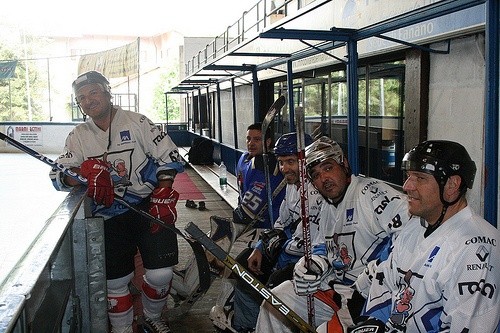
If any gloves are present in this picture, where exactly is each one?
[291,253,329,296]
[148,187,180,235]
[258,228,288,251]
[79,158,114,207]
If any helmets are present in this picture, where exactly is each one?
[72,70,109,92]
[273,132,314,156]
[401,140,477,189]
[305,136,344,181]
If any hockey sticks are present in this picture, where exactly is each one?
[261,95,286,230]
[184,220,318,333]
[240,128,329,234]
[0,131,211,290]
[295,107,316,330]
[161,121,328,321]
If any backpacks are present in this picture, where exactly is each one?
[184,138,214,165]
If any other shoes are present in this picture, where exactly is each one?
[142,313,172,333]
[186,200,197,208]
[209,306,255,333]
[199,201,205,210]
[171,268,190,297]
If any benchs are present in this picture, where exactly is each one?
[167,129,247,210]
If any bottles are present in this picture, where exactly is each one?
[219,162,229,192]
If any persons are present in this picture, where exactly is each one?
[347,140,500,333]
[254,135,412,333]
[169,122,324,333]
[50,71,186,333]
